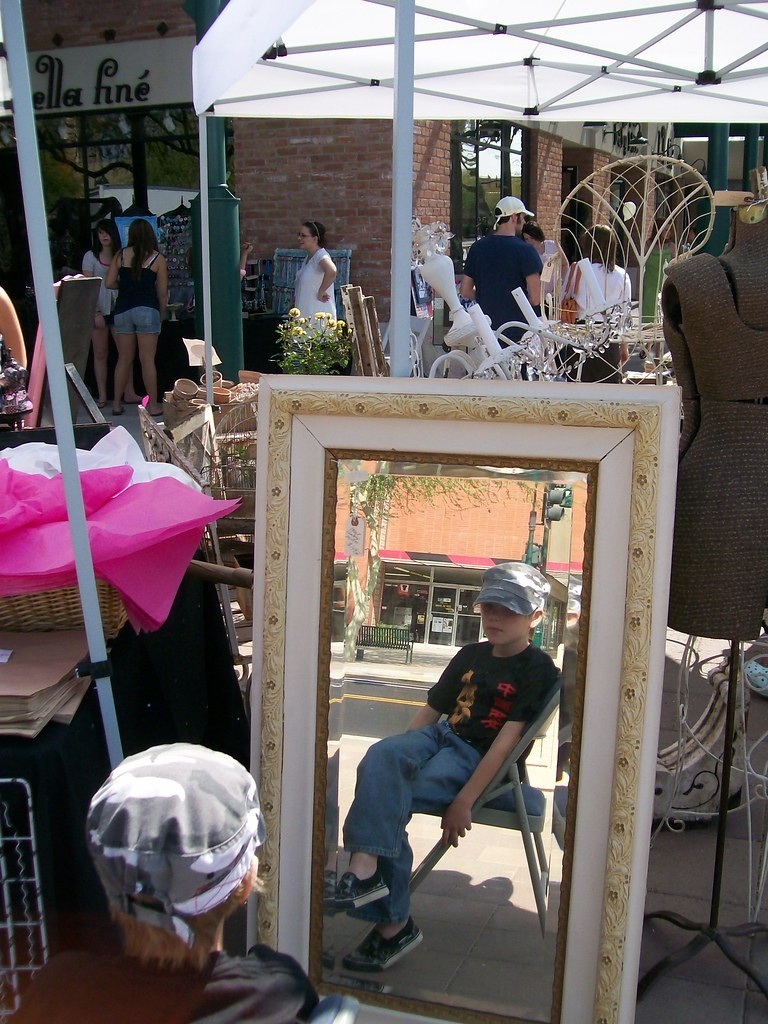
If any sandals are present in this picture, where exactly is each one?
[744,660,768,697]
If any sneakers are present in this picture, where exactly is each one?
[342,913,424,972]
[322,865,391,913]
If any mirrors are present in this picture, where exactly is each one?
[255,368,686,1024]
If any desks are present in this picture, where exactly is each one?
[89,314,283,404]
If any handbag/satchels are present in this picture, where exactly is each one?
[561,262,581,323]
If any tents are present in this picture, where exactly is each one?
[186,1,768,402]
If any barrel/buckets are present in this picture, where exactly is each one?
[357,649,364,659]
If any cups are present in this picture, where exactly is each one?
[645,361,653,372]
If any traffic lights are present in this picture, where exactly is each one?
[542,486,567,521]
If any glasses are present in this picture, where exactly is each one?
[297,233,314,238]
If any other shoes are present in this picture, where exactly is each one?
[112,407,123,415]
[149,410,163,416]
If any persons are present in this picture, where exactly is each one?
[0,742,319,1024]
[331,562,559,974]
[240,242,255,282]
[0,286,27,395]
[558,225,632,385]
[521,221,564,303]
[460,196,544,348]
[82,219,148,408]
[290,220,338,374]
[638,218,676,359]
[104,217,168,417]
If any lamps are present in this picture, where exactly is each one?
[653,143,687,165]
[582,121,607,129]
[681,158,707,175]
[409,217,634,382]
[602,122,647,146]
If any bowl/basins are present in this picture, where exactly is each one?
[171,371,235,405]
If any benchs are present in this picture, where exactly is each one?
[347,622,418,666]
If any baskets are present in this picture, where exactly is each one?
[0,578,130,641]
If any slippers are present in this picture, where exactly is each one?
[125,400,149,404]
[97,401,105,408]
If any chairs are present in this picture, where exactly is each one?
[341,281,431,376]
[411,684,562,942]
[552,155,711,386]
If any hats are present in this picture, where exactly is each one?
[472,563,552,615]
[84,743,269,949]
[494,196,535,230]
